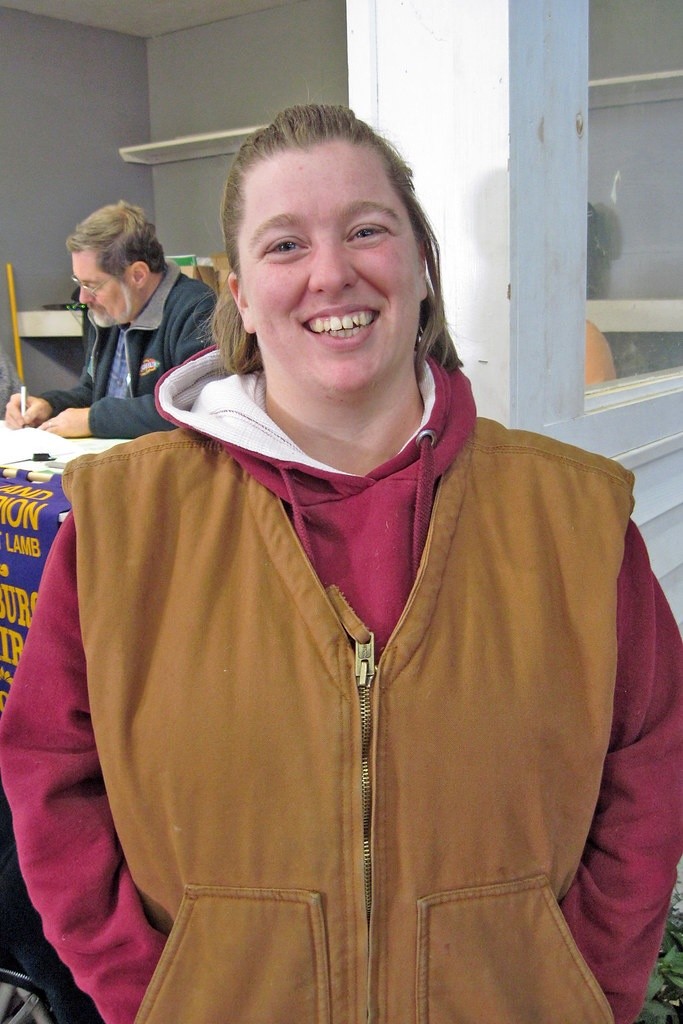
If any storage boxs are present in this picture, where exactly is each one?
[211,253,230,291]
[177,263,217,294]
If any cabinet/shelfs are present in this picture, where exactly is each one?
[17,68,683,339]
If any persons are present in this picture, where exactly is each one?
[0,102,683,1024]
[0,347,28,421]
[5,202,221,439]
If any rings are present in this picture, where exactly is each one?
[49,421,52,428]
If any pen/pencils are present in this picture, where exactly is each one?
[20,384,26,428]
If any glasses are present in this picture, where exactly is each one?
[70,272,116,297]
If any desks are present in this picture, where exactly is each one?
[0,437,135,715]
